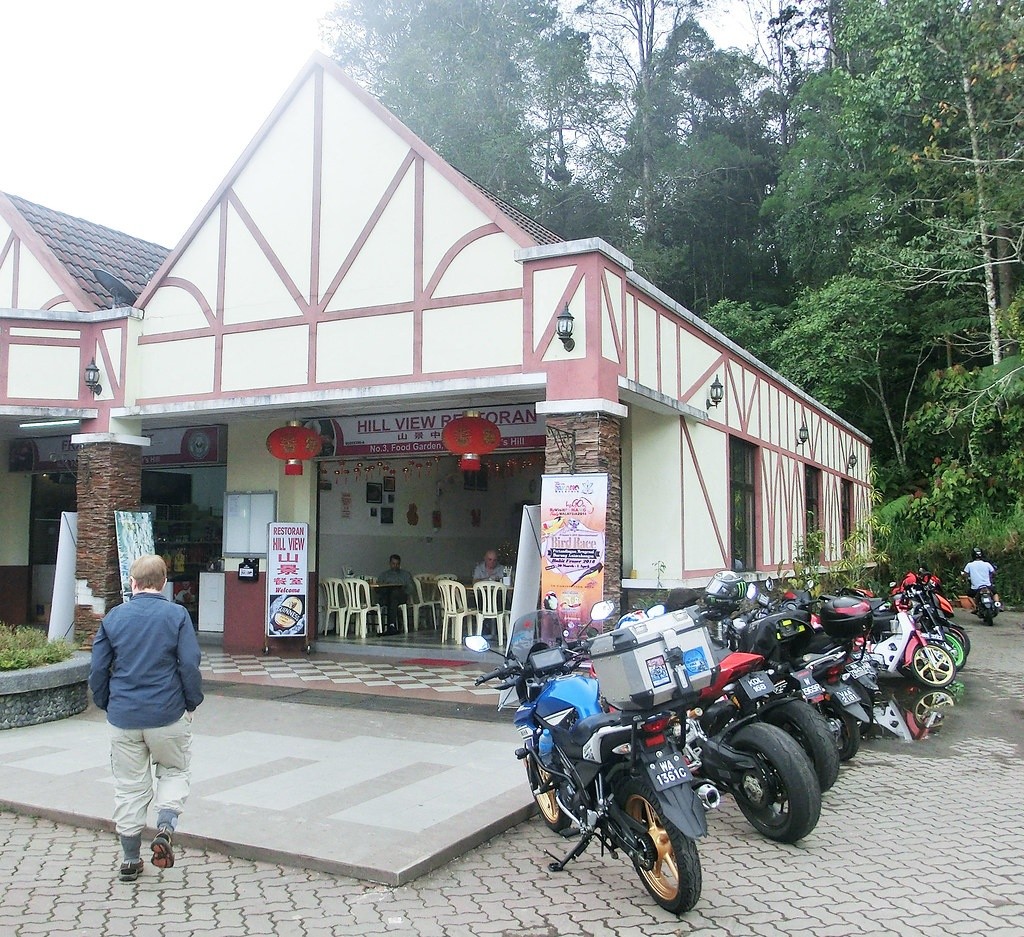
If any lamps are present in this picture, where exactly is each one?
[705,374,723,410]
[796,422,808,446]
[846,451,857,472]
[556,302,576,352]
[84,357,102,396]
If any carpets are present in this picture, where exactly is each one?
[400,657,478,667]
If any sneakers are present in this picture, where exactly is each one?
[151,833,174,868]
[119,857,144,880]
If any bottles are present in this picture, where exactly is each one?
[161,547,187,574]
[360,570,364,580]
[495,576,499,582]
[269,595,304,631]
[502,569,507,577]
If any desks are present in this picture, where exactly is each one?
[425,579,514,596]
[347,583,402,635]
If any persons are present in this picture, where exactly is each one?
[374,554,414,634]
[90,555,205,880]
[472,550,510,635]
[961,547,1004,613]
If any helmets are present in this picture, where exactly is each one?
[972,547,982,560]
[700,570,749,622]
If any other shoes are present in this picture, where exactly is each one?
[970,609,978,614]
[385,625,396,635]
[998,607,1005,612]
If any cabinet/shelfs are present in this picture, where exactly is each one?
[154,519,223,577]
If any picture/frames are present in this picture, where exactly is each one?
[464,464,488,491]
[381,507,393,524]
[384,476,396,493]
[366,482,383,504]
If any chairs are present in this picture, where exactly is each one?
[320,572,512,647]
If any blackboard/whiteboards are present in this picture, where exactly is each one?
[222,489,278,557]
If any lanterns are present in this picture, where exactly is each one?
[441,410,500,471]
[319,456,462,484]
[486,456,544,473]
[266,420,323,475]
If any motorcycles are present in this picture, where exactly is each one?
[591,570,878,844]
[462,599,726,914]
[859,560,972,688]
[961,566,1004,627]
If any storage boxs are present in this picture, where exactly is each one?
[586,609,720,712]
[818,596,873,639]
